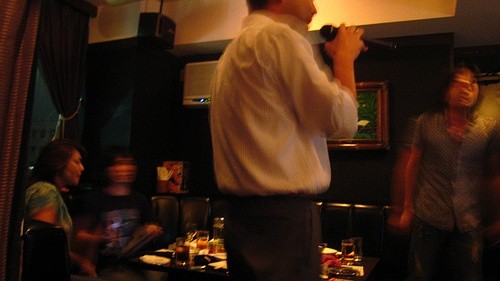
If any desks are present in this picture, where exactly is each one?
[126,238,380,281]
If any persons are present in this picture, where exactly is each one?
[24,140,98,281]
[211,0,364,281]
[398,61,487,281]
[74,145,164,267]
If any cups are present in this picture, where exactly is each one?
[158,180,169,192]
[341,239,355,261]
[354,237,362,261]
[175,237,190,266]
[196,230,209,249]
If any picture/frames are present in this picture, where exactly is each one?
[327,81,391,150]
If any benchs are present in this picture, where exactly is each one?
[151,196,411,261]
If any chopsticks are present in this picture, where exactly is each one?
[157,167,172,180]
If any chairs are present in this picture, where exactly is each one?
[17,207,71,281]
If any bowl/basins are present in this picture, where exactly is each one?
[153,250,174,258]
[189,250,199,261]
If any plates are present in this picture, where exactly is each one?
[139,255,171,266]
[321,248,342,255]
[194,253,227,269]
[329,265,364,276]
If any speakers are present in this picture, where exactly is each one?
[137,13,176,49]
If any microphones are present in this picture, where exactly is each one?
[320,25,397,50]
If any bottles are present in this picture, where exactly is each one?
[213,217,224,243]
